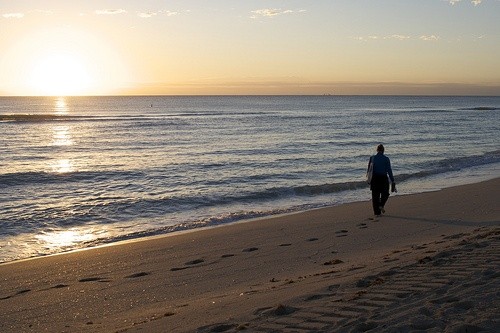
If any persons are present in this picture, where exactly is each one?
[367,143,395,216]
